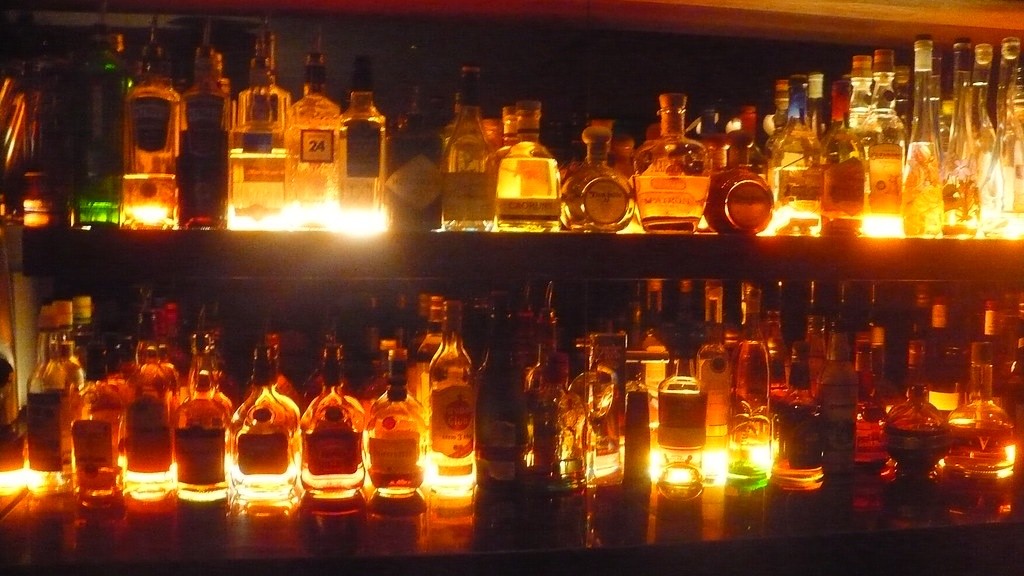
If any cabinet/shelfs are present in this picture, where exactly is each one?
[0,0,1024,576]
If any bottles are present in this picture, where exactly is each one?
[0,279,1024,521]
[0,28,1023,236]
